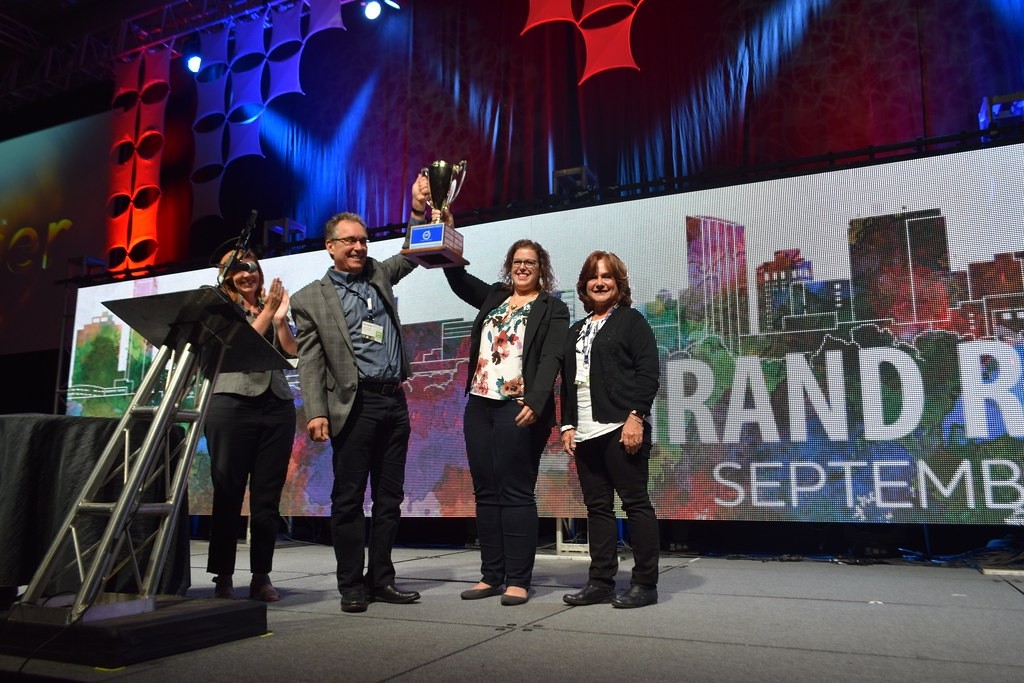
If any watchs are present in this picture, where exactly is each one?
[411,206,428,216]
[630,410,647,420]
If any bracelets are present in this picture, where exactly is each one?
[629,415,645,427]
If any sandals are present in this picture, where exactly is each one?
[215,585,238,600]
[249,583,281,601]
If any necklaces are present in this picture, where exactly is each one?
[511,292,528,310]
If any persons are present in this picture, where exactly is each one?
[558,249,662,610]
[430,206,571,605]
[198,249,300,602]
[290,173,431,613]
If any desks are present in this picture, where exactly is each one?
[0,413,191,609]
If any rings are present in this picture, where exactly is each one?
[531,416,534,419]
[283,301,286,304]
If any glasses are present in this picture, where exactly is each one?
[331,237,370,246]
[511,260,541,268]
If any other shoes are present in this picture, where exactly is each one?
[501,589,528,605]
[460,587,503,600]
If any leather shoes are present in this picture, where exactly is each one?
[340,589,367,611]
[368,584,421,602]
[613,586,657,608]
[563,584,616,605]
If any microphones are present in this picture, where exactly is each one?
[229,260,257,273]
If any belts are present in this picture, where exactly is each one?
[358,381,403,395]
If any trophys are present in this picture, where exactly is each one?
[399,160,471,269]
